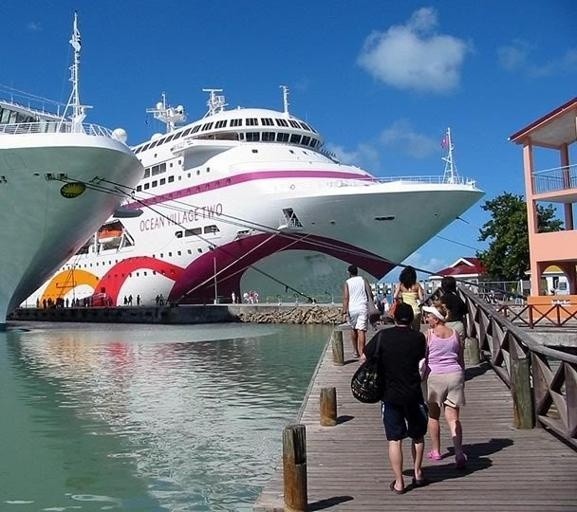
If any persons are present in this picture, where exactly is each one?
[341,264,373,358]
[35,293,166,308]
[231,289,260,304]
[368,284,395,324]
[441,276,469,372]
[394,266,424,330]
[418,302,467,465]
[358,303,427,493]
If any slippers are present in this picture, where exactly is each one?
[455,455,465,465]
[412,478,425,485]
[390,480,405,494]
[427,451,441,459]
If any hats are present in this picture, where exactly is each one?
[422,304,449,322]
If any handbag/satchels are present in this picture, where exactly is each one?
[351,355,385,402]
[389,300,402,320]
[422,328,433,380]
[367,301,380,324]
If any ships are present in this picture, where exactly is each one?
[15,79,491,310]
[1,4,150,340]
